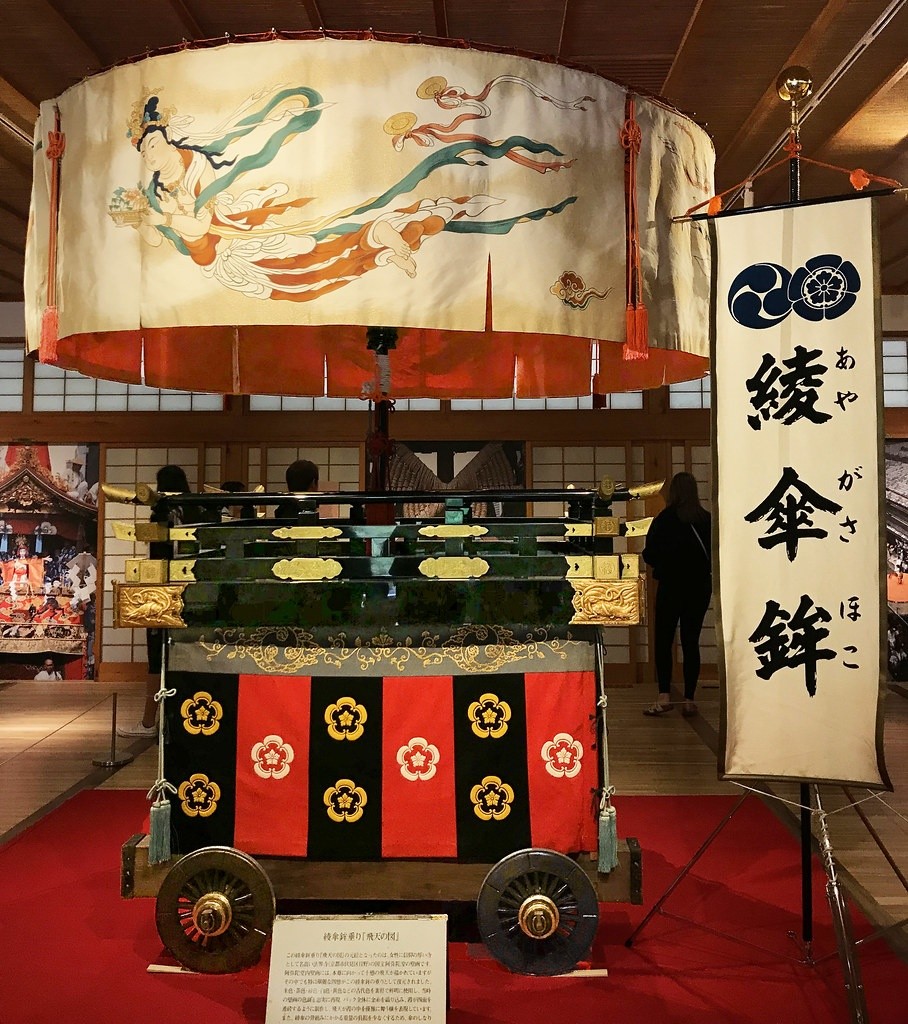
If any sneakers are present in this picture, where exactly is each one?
[117,721,158,739]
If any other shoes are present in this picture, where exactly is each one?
[643,704,674,715]
[682,705,697,716]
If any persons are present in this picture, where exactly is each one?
[115,466,202,737]
[34,658,63,681]
[65,455,98,504]
[641,470,712,716]
[274,459,322,517]
[222,480,254,519]
